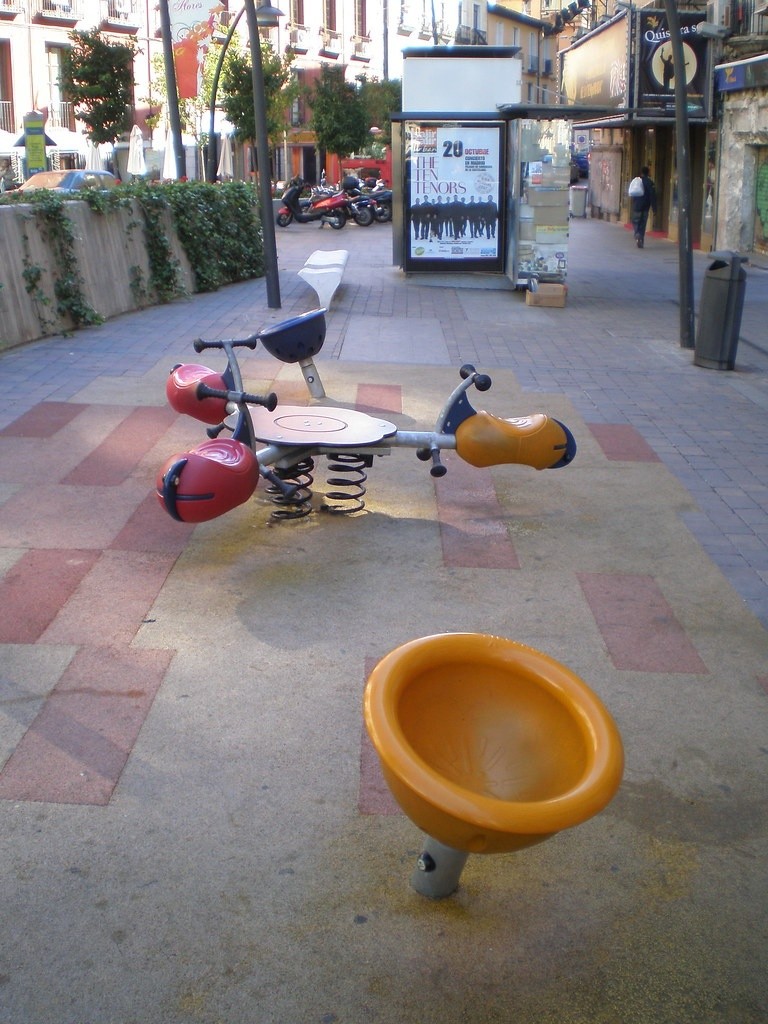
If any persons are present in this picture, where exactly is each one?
[632,166,657,249]
[411,195,498,242]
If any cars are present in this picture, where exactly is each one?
[570,156,581,182]
[2,169,125,197]
[576,150,587,177]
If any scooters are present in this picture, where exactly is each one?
[277,168,392,228]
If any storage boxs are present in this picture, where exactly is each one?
[519,164,571,242]
[526,284,567,308]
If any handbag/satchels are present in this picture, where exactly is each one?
[628,176,644,197]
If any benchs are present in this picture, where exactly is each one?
[297,249,350,312]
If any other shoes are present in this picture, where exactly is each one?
[636,237,643,248]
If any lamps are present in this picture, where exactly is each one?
[694,21,734,39]
[555,0,598,29]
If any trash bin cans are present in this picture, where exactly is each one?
[693,250,749,372]
[569,185,588,218]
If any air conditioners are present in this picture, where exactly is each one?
[289,30,307,46]
